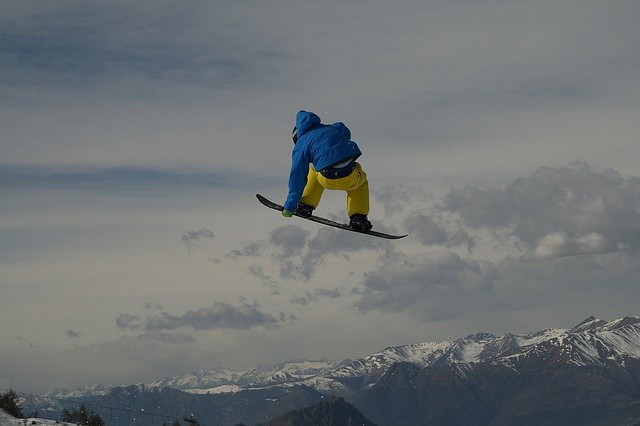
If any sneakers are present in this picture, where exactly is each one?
[350,215,371,231]
[296,203,313,217]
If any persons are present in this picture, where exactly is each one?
[283,110,373,230]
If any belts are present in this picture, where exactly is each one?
[320,172,351,179]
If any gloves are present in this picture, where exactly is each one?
[282,209,293,217]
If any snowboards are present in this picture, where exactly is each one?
[256,193,408,240]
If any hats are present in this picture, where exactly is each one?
[292,126,297,143]
